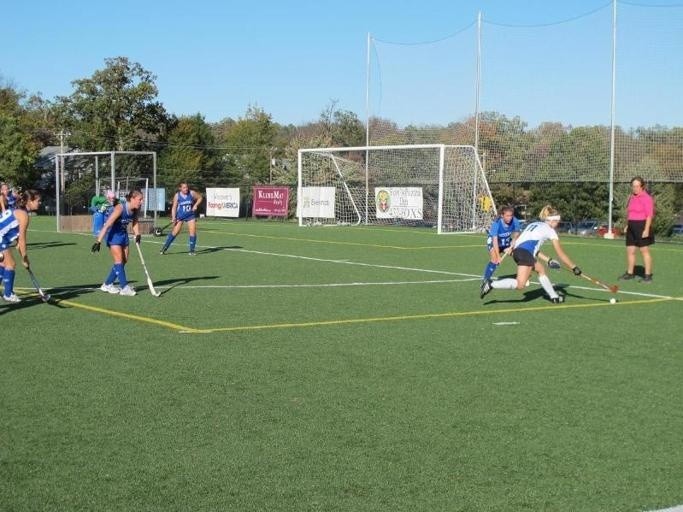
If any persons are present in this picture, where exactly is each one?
[90,188,144,296]
[159,180,203,256]
[479,203,582,303]
[480,203,524,291]
[616,176,656,284]
[0,189,43,304]
[0,181,23,213]
[90,183,127,237]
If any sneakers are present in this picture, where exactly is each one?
[119,284,136,296]
[640,273,653,284]
[553,294,565,304]
[618,272,635,281]
[479,279,492,298]
[3,293,21,302]
[100,282,120,294]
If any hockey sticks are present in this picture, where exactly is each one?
[157,210,193,234]
[134,242,162,297]
[14,242,53,303]
[495,231,518,266]
[560,264,618,293]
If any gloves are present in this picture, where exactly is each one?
[549,259,561,268]
[135,234,141,244]
[91,241,101,253]
[572,265,581,275]
